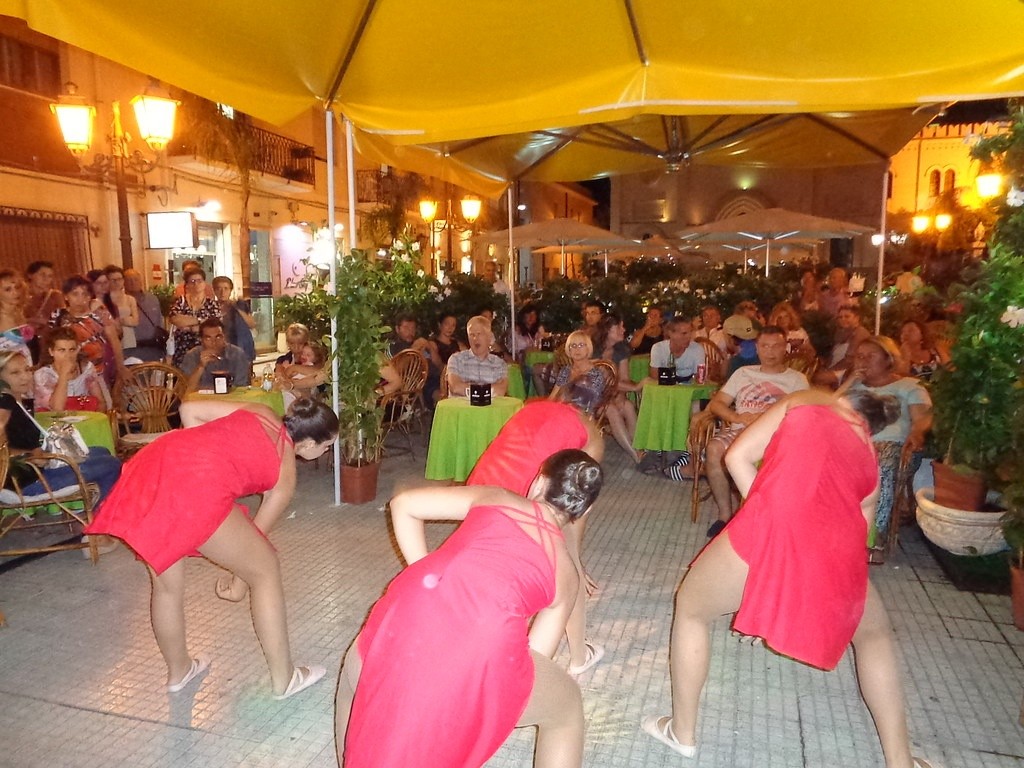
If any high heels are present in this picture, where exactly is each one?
[82,535,119,561]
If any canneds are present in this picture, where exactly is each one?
[696,363,705,384]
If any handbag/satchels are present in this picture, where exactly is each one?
[153,326,169,346]
[63,393,100,415]
[41,421,89,470]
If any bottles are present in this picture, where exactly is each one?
[666,354,675,368]
[263,364,273,392]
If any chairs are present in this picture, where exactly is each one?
[1,333,950,565]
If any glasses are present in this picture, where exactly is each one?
[570,343,587,349]
[188,278,203,284]
[202,335,225,342]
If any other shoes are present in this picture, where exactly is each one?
[868,546,886,564]
[640,714,696,759]
[899,485,916,523]
[707,519,727,540]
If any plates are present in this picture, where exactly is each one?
[59,416,90,422]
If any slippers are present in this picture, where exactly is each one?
[672,455,701,466]
[271,663,326,702]
[663,465,700,482]
[634,451,662,474]
[165,654,212,692]
[566,641,605,676]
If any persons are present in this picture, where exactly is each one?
[81,394,342,701]
[637,385,940,768]
[332,447,607,768]
[462,359,607,675]
[0,258,983,563]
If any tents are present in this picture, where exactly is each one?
[336,102,960,335]
[0,0,1024,516]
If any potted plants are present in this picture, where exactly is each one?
[329,250,390,505]
[1006,519,1024,628]
[915,98,1024,509]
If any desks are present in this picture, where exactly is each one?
[190,385,289,428]
[0,404,116,542]
[525,348,559,367]
[628,378,726,459]
[625,353,651,382]
[493,359,527,400]
[421,398,521,486]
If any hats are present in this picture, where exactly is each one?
[723,314,759,341]
[867,335,901,372]
[0,330,34,370]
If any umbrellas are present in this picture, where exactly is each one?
[482,205,879,255]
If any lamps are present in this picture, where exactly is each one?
[292,219,308,226]
